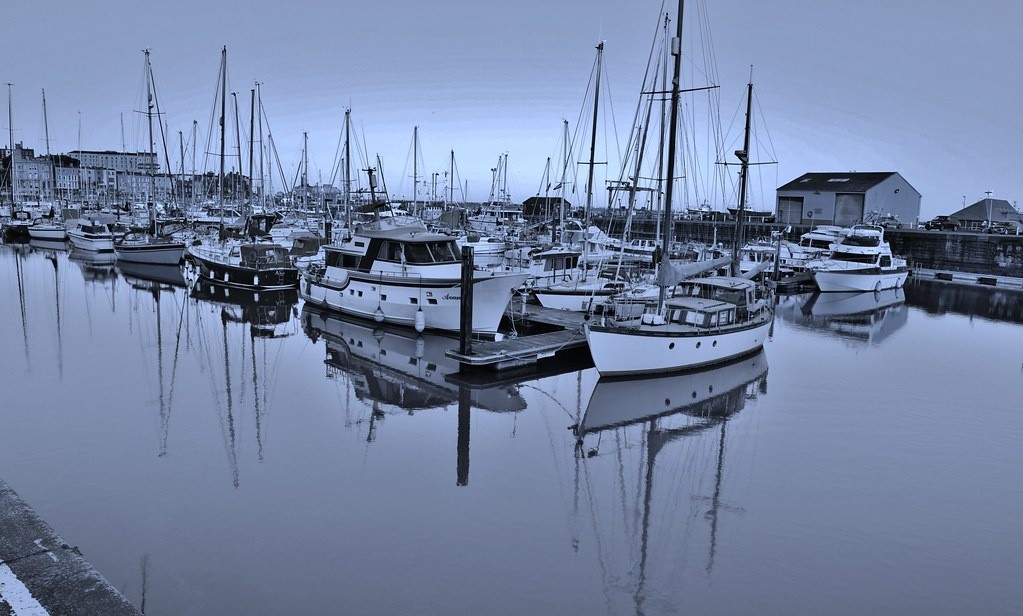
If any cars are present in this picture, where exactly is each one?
[989,221,1020,235]
[679,212,701,220]
[569,206,587,218]
[872,216,903,229]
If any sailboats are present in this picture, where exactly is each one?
[0,243,296,489]
[0,0,774,377]
[569,349,771,616]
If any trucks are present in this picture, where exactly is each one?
[702,212,728,221]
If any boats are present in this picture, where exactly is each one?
[776,221,846,274]
[299,301,528,445]
[66,215,122,252]
[799,288,907,348]
[806,224,909,293]
[727,189,772,221]
[293,224,531,337]
[685,199,720,214]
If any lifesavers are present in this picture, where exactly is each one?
[306,263,320,275]
[248,251,257,262]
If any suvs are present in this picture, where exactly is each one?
[925,216,961,231]
